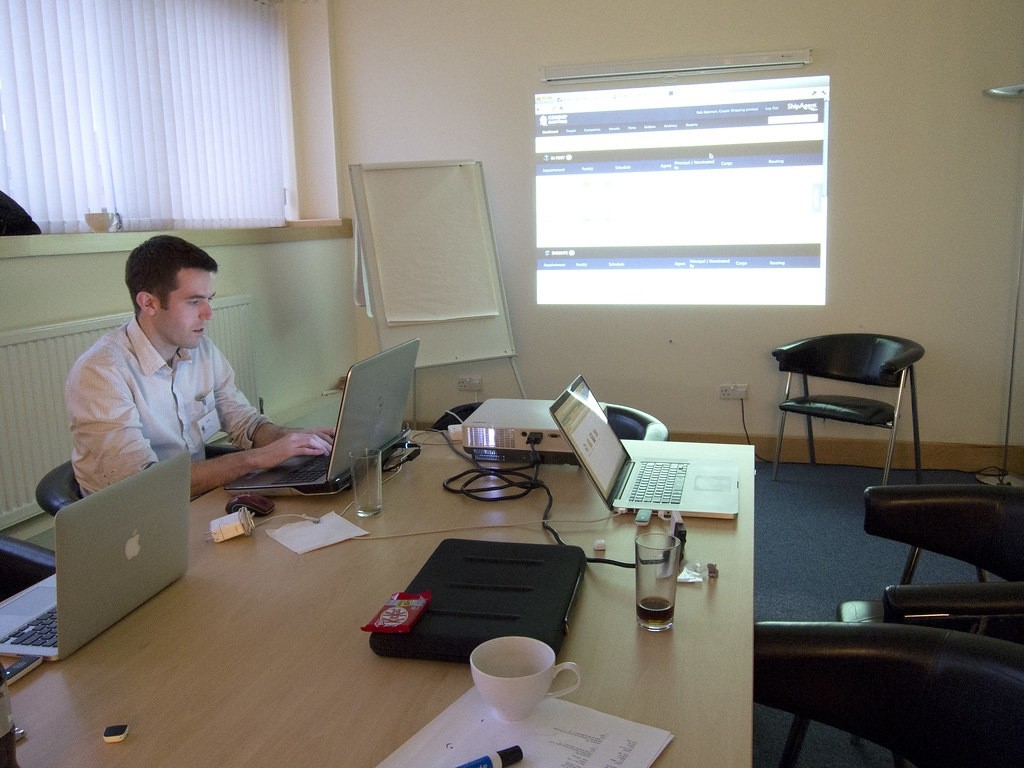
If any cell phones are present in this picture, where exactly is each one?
[0,656,43,686]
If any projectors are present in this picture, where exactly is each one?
[462,398,608,467]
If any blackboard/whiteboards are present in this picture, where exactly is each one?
[348,160,518,370]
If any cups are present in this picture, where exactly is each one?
[635,533,681,631]
[349,449,383,517]
[469,637,581,719]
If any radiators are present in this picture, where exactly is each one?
[0,292,261,530]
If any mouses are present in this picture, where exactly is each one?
[225,493,274,517]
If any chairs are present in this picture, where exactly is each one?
[748,622,1024,768]
[0,532,56,600]
[35,458,87,517]
[430,402,668,442]
[772,334,925,488]
[837,485,1024,744]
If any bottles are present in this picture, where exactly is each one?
[0,662,23,768]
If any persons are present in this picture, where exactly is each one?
[63,235,336,498]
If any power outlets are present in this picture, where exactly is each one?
[719,384,748,399]
[455,375,482,391]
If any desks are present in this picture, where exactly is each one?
[0,430,756,768]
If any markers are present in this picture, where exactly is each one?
[454,745,523,768]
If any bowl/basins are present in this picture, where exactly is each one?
[84,212,114,232]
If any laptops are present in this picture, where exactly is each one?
[548,374,739,519]
[0,450,192,662]
[224,338,421,497]
